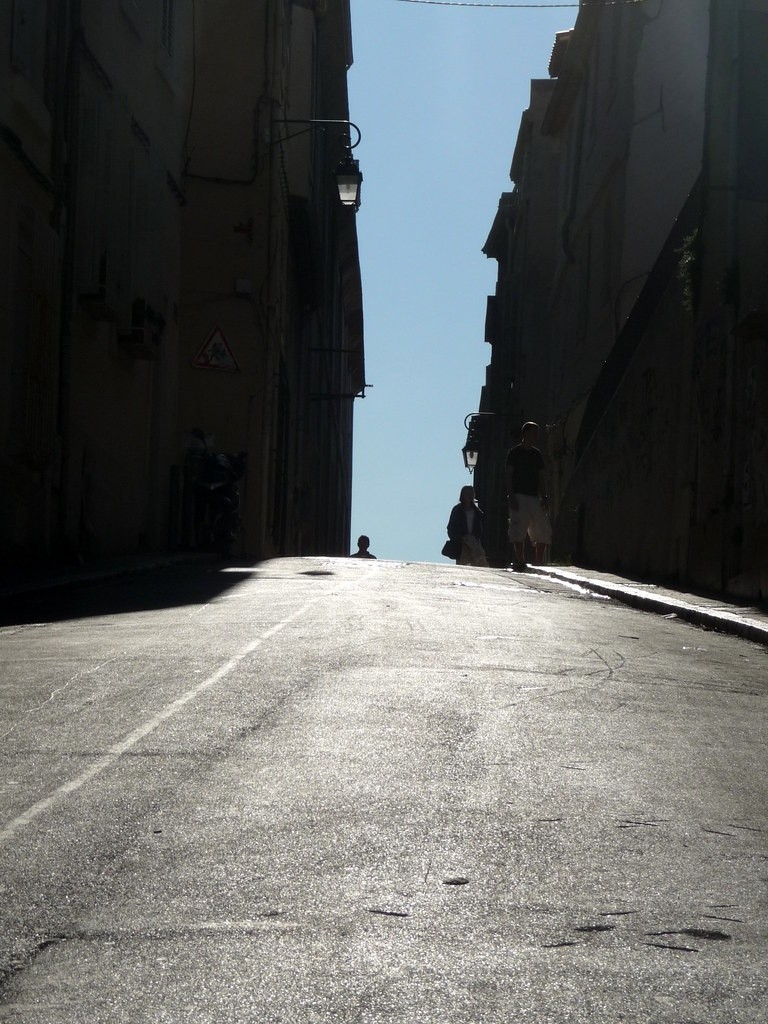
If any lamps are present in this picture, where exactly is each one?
[272,117,363,213]
[463,413,482,467]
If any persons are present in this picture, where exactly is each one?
[506,422,551,573]
[349,536,376,559]
[448,486,488,566]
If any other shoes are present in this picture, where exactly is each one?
[513,563,527,572]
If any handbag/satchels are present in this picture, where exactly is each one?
[441,540,461,559]
